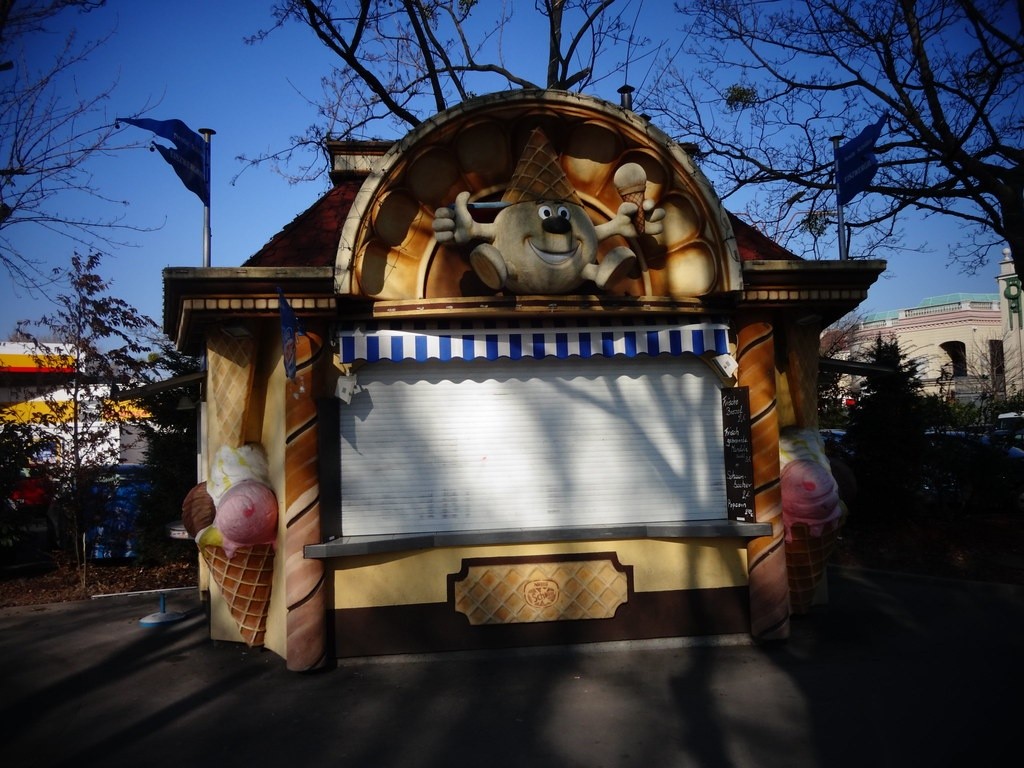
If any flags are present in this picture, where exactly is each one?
[835,111,888,206]
[115,117,209,205]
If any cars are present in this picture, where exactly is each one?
[819,427,1024,468]
[0,461,55,512]
[46,463,165,561]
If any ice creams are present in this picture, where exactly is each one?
[781,427,844,610]
[183,443,278,649]
[614,163,647,238]
